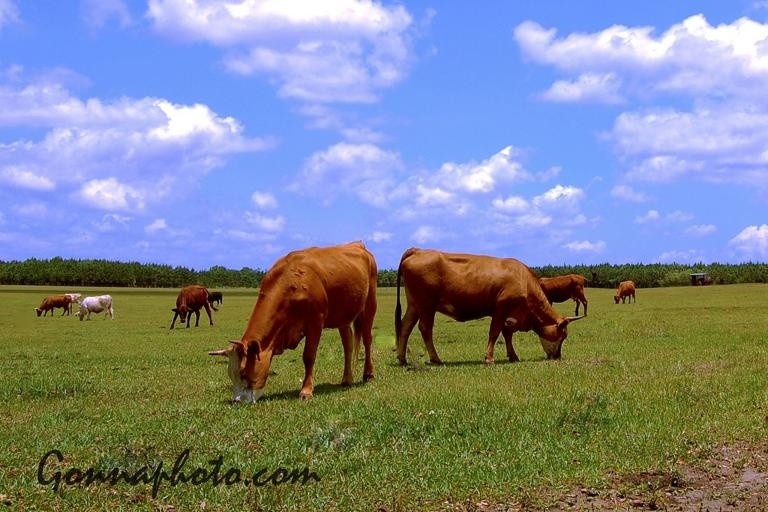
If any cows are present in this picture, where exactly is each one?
[209,292,223,305]
[34,295,73,317]
[538,274,588,317]
[614,280,636,304]
[394,247,585,367]
[207,239,378,406]
[170,284,213,330]
[65,293,81,304]
[75,294,114,321]
